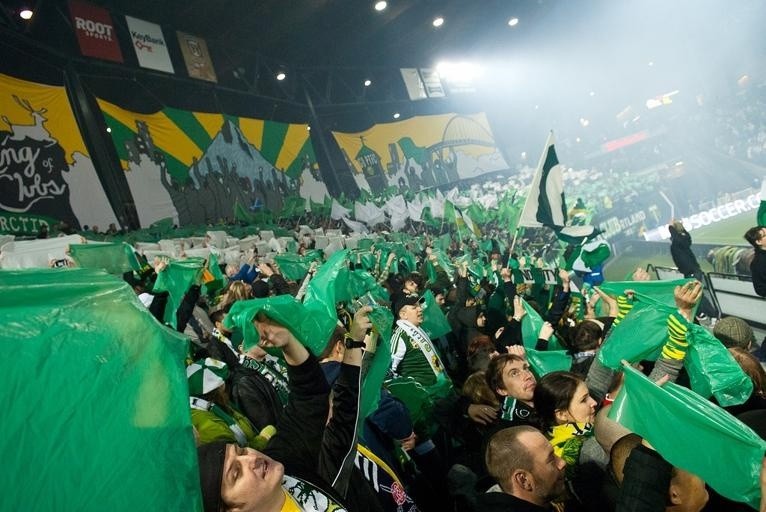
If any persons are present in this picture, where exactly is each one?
[32,75,765,511]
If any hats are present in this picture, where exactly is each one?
[186,357,231,396]
[713,316,759,349]
[393,292,425,316]
[583,265,604,285]
[198,439,227,512]
